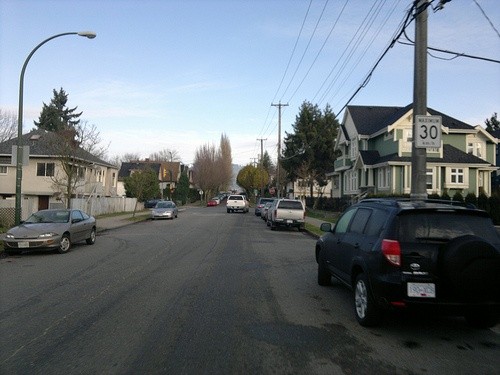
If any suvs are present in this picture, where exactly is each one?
[315,197,500,330]
[226,194,250,213]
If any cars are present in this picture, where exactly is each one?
[255,198,306,232]
[207,191,232,206]
[2,209,97,253]
[153,201,179,219]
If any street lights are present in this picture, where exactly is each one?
[15,31,96,225]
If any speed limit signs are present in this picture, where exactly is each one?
[415,115,441,148]
